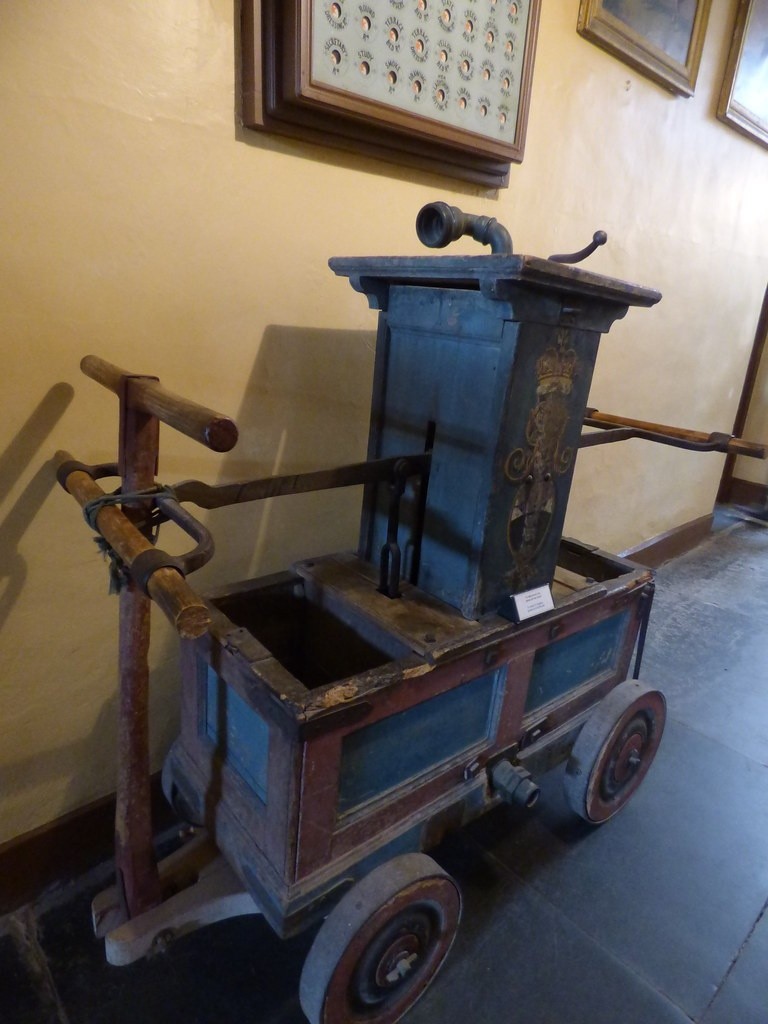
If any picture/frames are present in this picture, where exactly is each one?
[716,0,768,154]
[575,1,714,99]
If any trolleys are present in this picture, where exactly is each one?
[51,198,768,1024]
